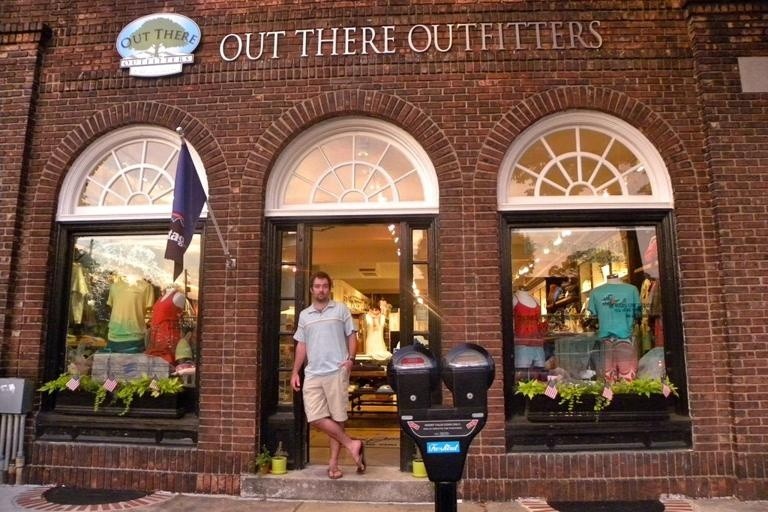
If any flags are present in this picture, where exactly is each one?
[164,140,208,281]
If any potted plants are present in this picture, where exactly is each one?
[516,378,679,425]
[39,372,187,419]
[255,444,270,474]
[269,440,288,474]
[412,455,428,478]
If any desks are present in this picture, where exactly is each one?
[351,369,398,415]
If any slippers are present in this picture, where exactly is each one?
[356,440,366,474]
[327,466,343,478]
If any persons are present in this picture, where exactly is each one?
[588,272,643,381]
[145,287,188,364]
[289,271,368,479]
[365,307,388,355]
[511,289,546,369]
[105,268,156,354]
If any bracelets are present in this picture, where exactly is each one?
[347,354,355,362]
